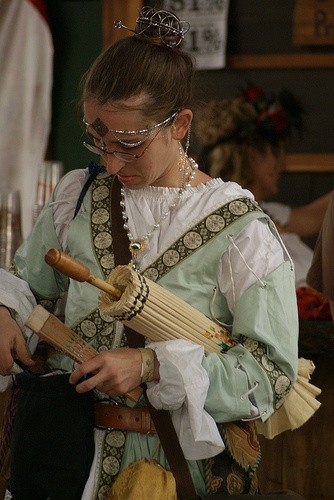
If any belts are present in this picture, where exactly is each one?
[93,403,158,435]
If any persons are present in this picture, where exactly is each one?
[0,3,321,500]
[0,1,53,211]
[188,83,334,287]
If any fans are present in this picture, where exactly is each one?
[238,80,304,141]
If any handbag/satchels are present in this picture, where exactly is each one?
[203,417,263,496]
[8,358,98,500]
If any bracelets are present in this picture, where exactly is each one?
[137,348,155,382]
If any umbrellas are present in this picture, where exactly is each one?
[45,248,321,440]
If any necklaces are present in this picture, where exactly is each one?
[120,156,198,274]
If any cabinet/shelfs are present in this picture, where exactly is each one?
[102,0,334,173]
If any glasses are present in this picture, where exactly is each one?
[80,120,170,164]
